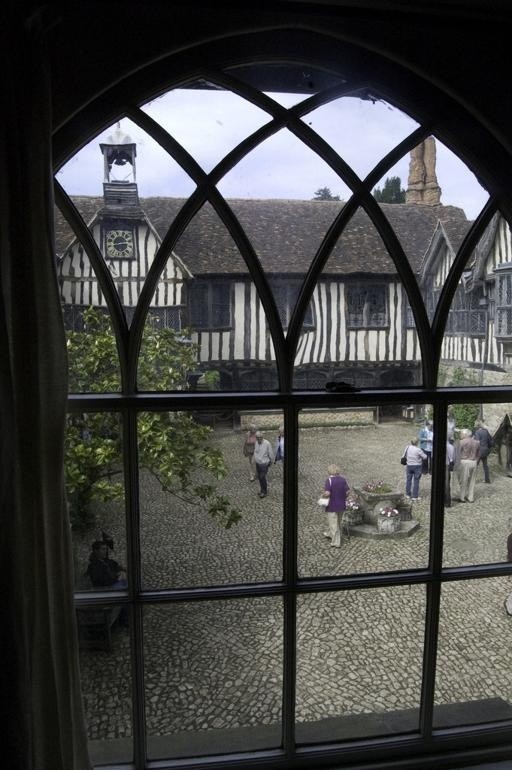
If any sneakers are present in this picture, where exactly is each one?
[257,491,267,498]
[445,496,475,508]
[249,474,256,482]
[405,494,423,501]
[323,531,332,539]
[330,541,341,548]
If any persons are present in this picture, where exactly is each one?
[274,426,284,462]
[252,430,274,497]
[243,423,257,481]
[317,463,350,548]
[401,403,492,508]
[86,540,143,627]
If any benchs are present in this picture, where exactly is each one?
[74,566,128,653]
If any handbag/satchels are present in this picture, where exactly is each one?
[317,497,331,507]
[400,457,408,466]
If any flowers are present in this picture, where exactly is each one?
[362,479,392,494]
[344,499,360,512]
[379,506,399,518]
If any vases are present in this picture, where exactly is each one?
[343,510,363,525]
[378,517,401,532]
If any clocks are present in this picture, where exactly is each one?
[103,226,137,261]
